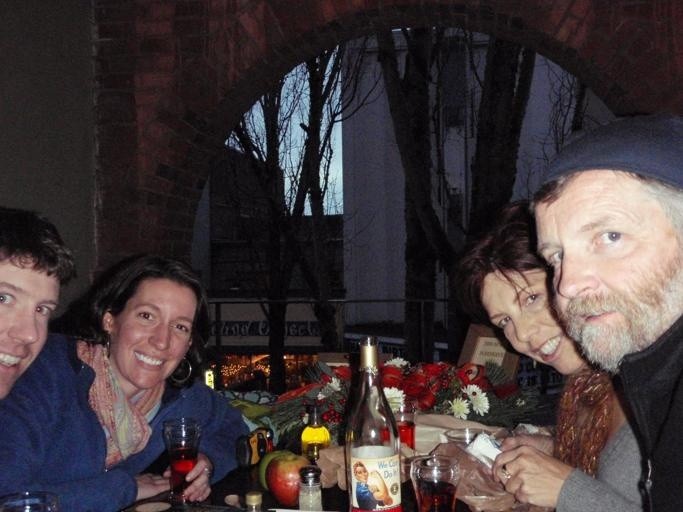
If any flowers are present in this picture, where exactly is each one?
[269,356,549,445]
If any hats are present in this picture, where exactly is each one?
[534,111,683,203]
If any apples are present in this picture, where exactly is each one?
[266,455,310,507]
[257,450,295,492]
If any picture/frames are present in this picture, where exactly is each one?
[457,323,522,384]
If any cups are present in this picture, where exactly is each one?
[444,427,491,444]
[2,492,58,511]
[409,453,461,511]
[160,416,203,511]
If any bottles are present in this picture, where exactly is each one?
[345,334,404,512]
[245,490,264,511]
[299,402,331,464]
[297,464,325,511]
